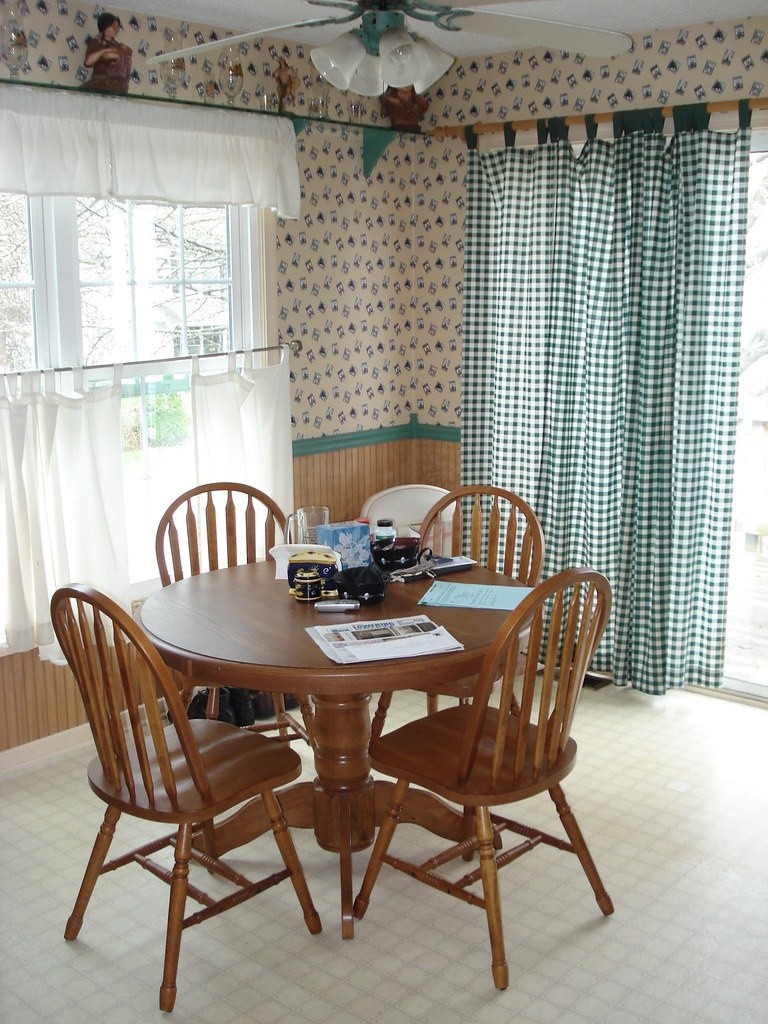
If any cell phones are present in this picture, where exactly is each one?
[315,599,361,612]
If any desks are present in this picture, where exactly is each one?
[136,559,544,941]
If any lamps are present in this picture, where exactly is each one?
[309,10,454,98]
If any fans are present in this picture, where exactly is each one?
[144,1,634,66]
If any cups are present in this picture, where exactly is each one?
[283,505,330,544]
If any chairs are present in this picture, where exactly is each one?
[49,583,321,1012]
[154,482,316,750]
[352,568,615,992]
[371,485,545,746]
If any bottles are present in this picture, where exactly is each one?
[293,569,322,602]
[373,519,397,543]
